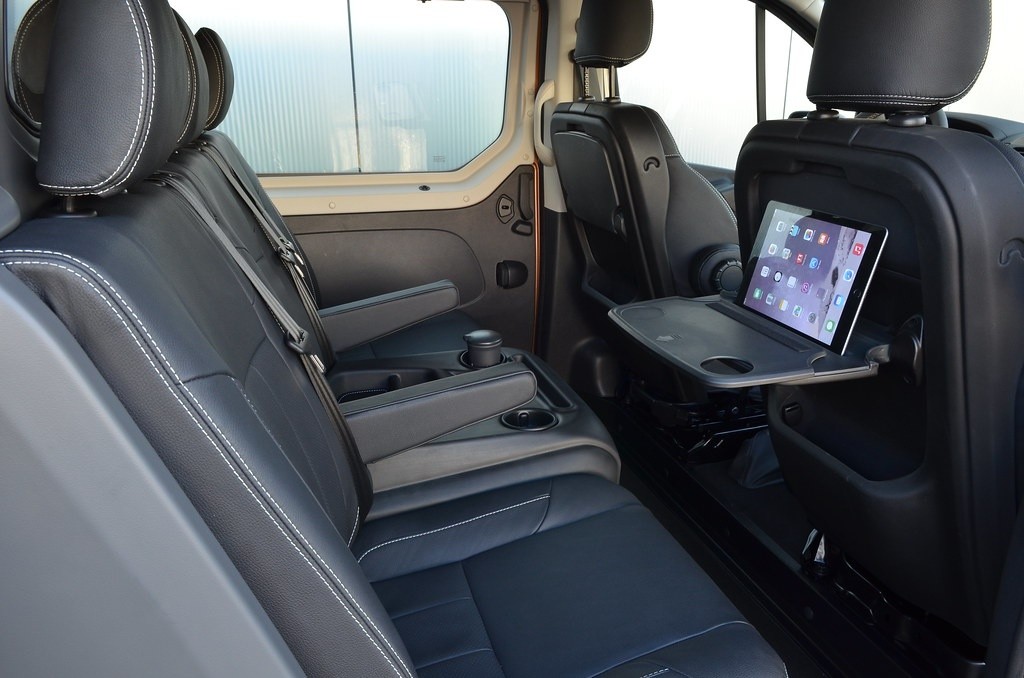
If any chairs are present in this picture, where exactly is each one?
[734,0,1024,677]
[0,0,788,678]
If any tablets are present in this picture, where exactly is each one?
[734,200,888,357]
